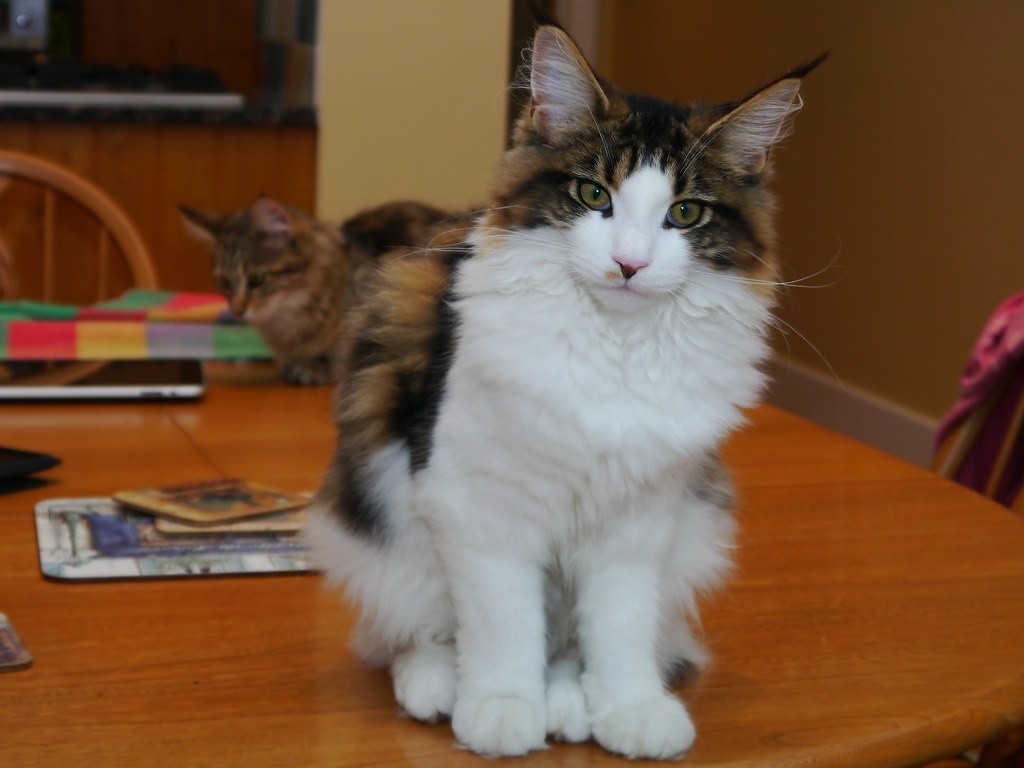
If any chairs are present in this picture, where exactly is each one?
[1,151,153,315]
[928,288,1024,765]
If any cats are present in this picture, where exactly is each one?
[175,199,466,388]
[306,5,828,759]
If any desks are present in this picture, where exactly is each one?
[0,351,1024,768]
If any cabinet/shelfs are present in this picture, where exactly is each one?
[0,107,316,305]
